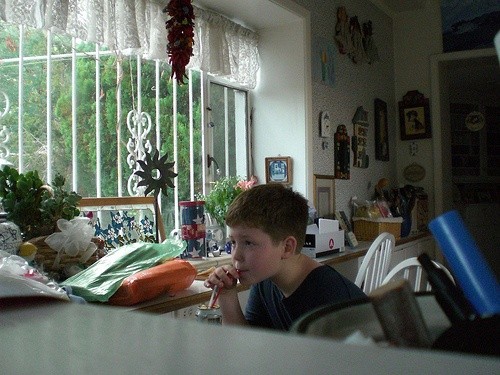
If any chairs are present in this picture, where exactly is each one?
[381,256,457,292]
[354,231,396,296]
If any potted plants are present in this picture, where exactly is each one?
[0,166,83,243]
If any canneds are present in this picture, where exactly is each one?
[195,303,222,327]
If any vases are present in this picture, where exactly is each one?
[211,218,228,251]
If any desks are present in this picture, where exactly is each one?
[0,298,499,375]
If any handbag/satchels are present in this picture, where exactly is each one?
[108,259,198,305]
[59,238,187,301]
[0,249,70,301]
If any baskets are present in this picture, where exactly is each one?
[352,217,403,242]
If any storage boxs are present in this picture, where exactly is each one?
[352,216,403,242]
[301,218,346,260]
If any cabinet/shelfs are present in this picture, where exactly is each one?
[93,229,436,325]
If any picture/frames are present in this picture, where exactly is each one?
[313,174,336,219]
[77,198,166,253]
[265,156,292,185]
[398,90,431,141]
[374,97,389,161]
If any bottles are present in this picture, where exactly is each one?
[179,201,206,260]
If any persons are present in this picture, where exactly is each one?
[204,182,367,332]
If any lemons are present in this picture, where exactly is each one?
[19,242,37,256]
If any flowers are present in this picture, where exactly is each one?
[195,168,251,228]
